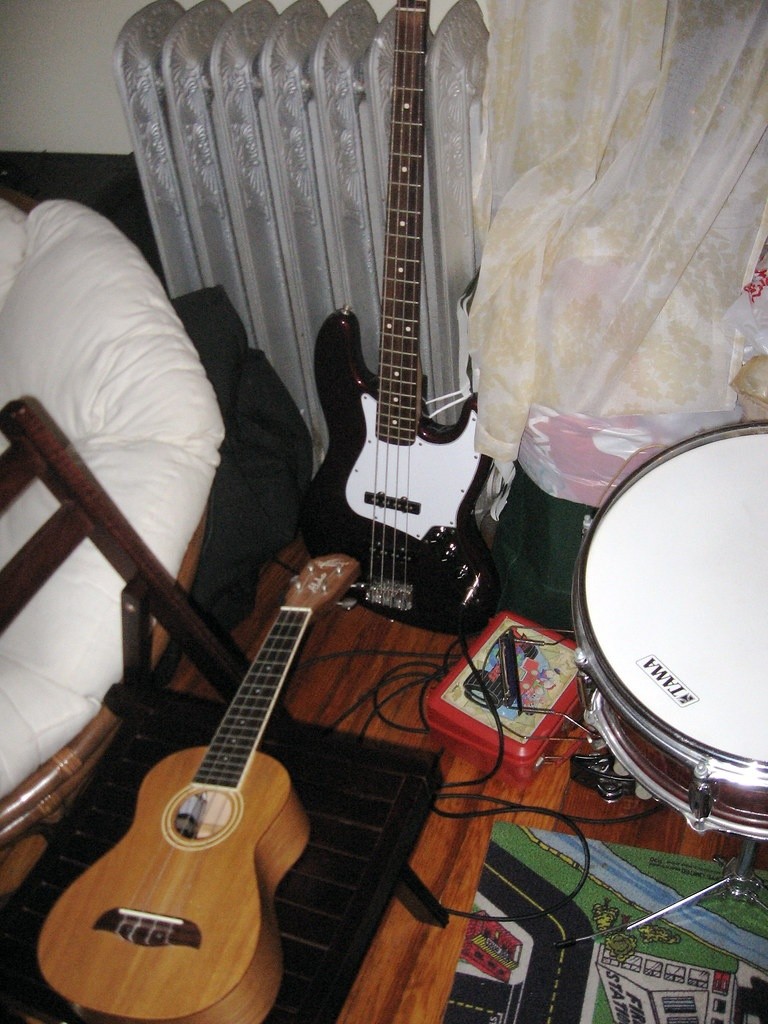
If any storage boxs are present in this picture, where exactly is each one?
[426,610,584,782]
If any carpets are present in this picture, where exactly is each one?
[443,818,767,1024]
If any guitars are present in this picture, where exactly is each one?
[31,549,363,1023]
[299,2,507,641]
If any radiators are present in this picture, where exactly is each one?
[111,0,489,480]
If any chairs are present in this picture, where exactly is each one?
[0,395,451,1024]
[0,185,226,856]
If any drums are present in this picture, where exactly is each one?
[562,415,768,851]
